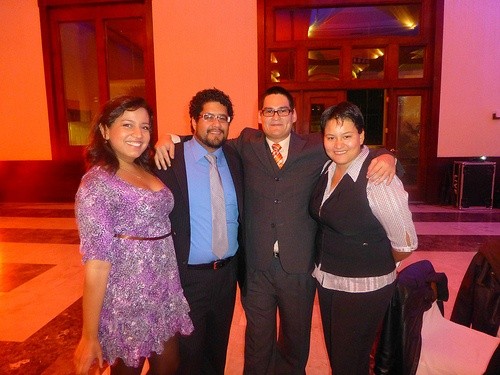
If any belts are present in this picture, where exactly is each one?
[113,231,173,241]
[187,257,230,271]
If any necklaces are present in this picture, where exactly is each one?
[119,163,144,179]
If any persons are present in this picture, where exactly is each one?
[149,88,244,375]
[309,102,418,375]
[72,95,196,375]
[154,85,398,375]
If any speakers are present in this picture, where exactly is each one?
[450,160,496,208]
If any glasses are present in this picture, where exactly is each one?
[261,107,294,116]
[193,113,234,122]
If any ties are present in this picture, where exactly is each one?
[271,143,285,169]
[204,153,228,260]
